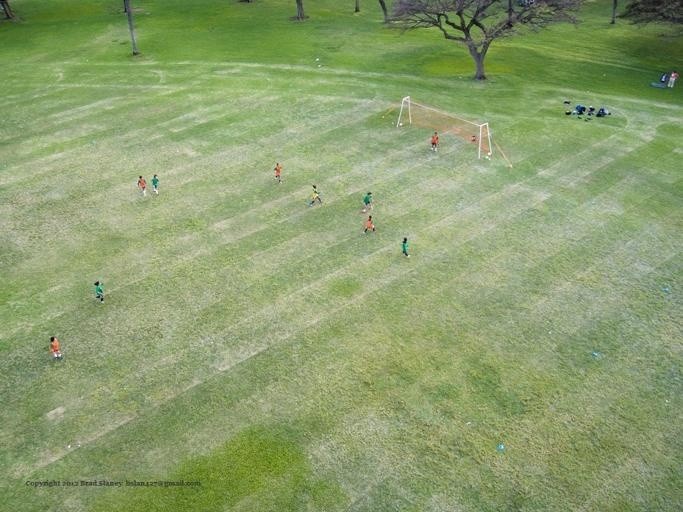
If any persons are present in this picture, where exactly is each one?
[668,69,678,88]
[574,103,587,117]
[586,105,595,116]
[596,108,612,119]
[429,130,439,152]
[361,192,375,213]
[308,184,322,206]
[150,175,160,197]
[272,163,282,184]
[400,237,412,258]
[361,216,376,235]
[49,337,63,360]
[93,281,105,303]
[658,72,669,84]
[136,175,148,198]
